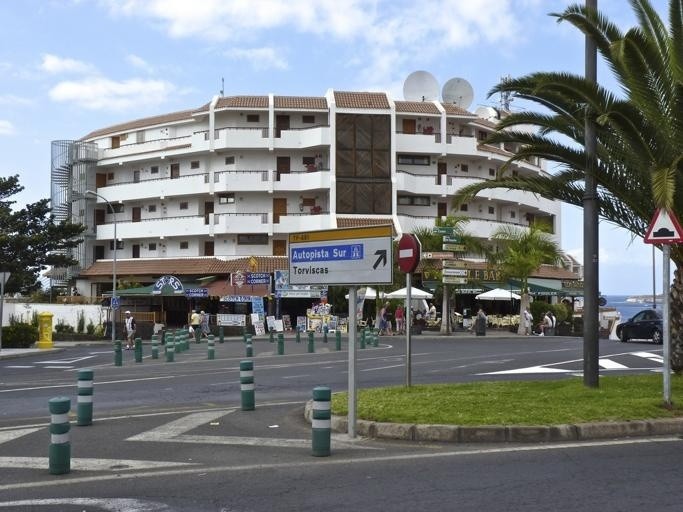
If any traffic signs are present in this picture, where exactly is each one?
[424,251,454,259]
[441,234,469,286]
[289,236,393,286]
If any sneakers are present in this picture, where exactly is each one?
[539,332,544,336]
[125,347,135,350]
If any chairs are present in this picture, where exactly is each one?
[488,314,521,332]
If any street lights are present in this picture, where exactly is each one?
[85,190,117,344]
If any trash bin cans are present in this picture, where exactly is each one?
[35,313,53,348]
[560,320,571,336]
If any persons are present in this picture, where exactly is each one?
[427,303,437,321]
[189,310,200,340]
[536,312,552,336]
[199,311,209,338]
[384,301,394,335]
[523,305,530,336]
[393,305,427,334]
[545,311,556,328]
[122,310,135,350]
[378,304,391,336]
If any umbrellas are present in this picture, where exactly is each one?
[474,287,522,302]
[344,286,385,300]
[385,286,433,300]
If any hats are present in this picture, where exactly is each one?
[192,310,196,312]
[201,311,205,314]
[125,310,131,314]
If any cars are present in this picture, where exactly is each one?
[615,308,663,345]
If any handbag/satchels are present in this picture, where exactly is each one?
[189,325,194,333]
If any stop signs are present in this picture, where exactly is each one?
[397,233,419,274]
